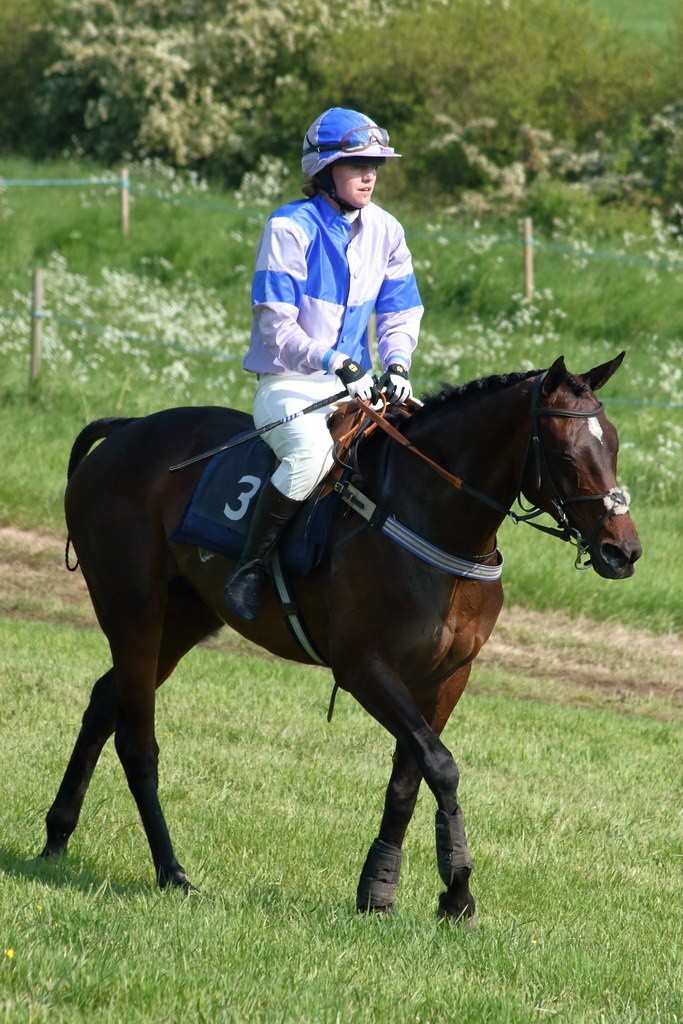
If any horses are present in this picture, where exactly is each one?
[36,350,643,929]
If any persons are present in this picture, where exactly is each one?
[224,107,423,623]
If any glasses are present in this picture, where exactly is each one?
[340,127,390,153]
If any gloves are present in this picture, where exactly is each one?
[331,353,378,406]
[377,356,413,405]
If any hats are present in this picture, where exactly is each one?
[301,107,402,176]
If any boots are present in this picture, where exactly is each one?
[223,470,310,622]
